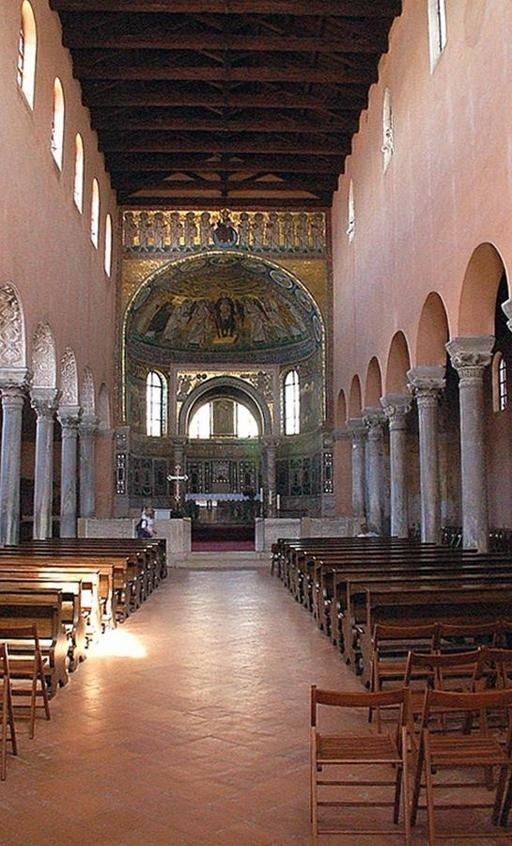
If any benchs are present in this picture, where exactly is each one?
[270,537,512,620]
[0,538,167,701]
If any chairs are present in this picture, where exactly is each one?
[0,620,51,784]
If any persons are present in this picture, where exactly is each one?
[135,507,157,540]
[122,210,326,256]
[140,290,306,346]
